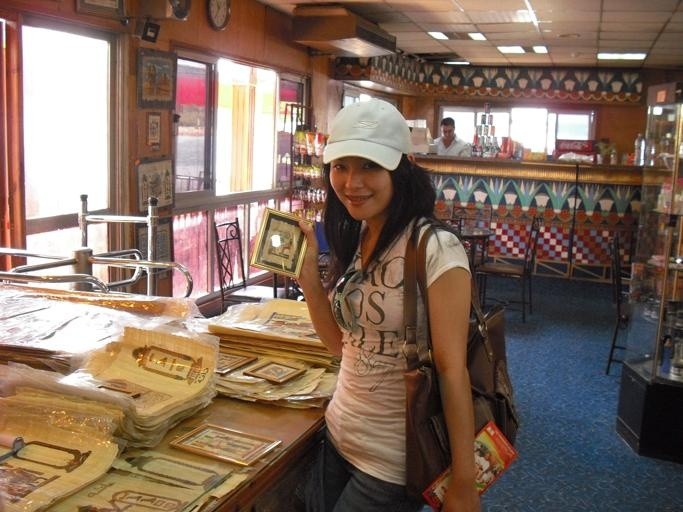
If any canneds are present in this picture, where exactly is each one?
[474,102,500,152]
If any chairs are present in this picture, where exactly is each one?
[475,214,544,324]
[213,217,294,313]
[604,230,667,375]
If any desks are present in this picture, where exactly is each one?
[452,225,496,305]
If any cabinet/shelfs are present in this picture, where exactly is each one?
[615,80,683,465]
[288,105,329,219]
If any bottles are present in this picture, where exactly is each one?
[633,133,645,168]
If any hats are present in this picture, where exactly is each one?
[323,98,415,171]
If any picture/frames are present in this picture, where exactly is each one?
[248,207,314,279]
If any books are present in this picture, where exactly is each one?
[420,418,520,512]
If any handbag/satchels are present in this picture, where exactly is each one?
[402,218,521,500]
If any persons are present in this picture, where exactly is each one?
[430,116,471,156]
[289,97,481,512]
[477,450,492,478]
